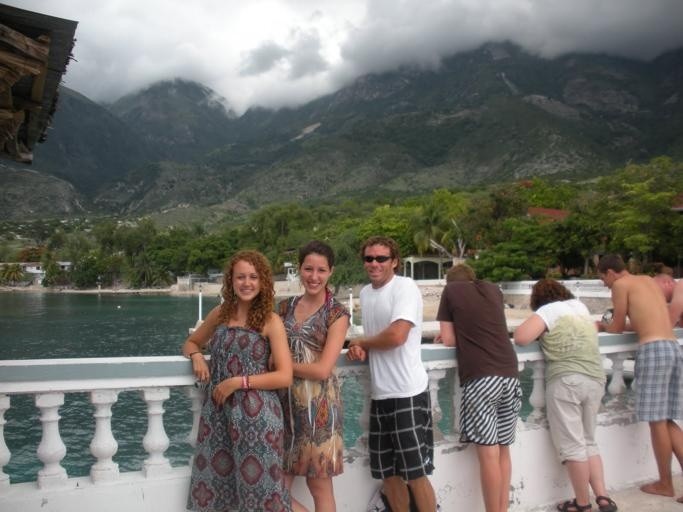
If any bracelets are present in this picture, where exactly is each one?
[187,351,202,359]
[242,374,250,392]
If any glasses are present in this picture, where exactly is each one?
[366,256,393,263]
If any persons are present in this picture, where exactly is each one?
[182,250,294,512]
[592,252,683,505]
[432,263,523,511]
[654,273,683,329]
[513,279,618,511]
[267,238,351,512]
[346,236,438,512]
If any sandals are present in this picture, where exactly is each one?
[557,498,592,512]
[596,496,617,512]
